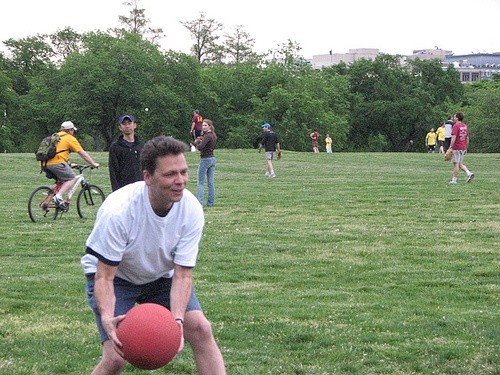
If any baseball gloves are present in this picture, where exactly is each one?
[277,151,282,159]
[444,147,453,162]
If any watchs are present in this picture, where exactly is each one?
[450,146,453,148]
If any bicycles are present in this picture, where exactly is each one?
[27,165,105,223]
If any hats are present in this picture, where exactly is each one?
[61,121,77,130]
[262,124,270,128]
[119,114,135,123]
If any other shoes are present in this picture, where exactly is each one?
[449,181,456,184]
[269,174,276,178]
[265,172,269,175]
[466,174,474,182]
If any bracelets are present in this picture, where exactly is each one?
[175,317,184,324]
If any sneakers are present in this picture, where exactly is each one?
[52,195,65,209]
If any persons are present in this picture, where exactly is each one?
[41,121,99,206]
[80,136,228,375]
[108,113,147,192]
[311,129,319,153]
[190,110,216,208]
[258,123,282,178]
[325,134,333,153]
[424,112,475,185]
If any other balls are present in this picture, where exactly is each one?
[145,108,149,111]
[115,302,181,371]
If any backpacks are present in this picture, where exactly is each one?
[36,133,69,161]
